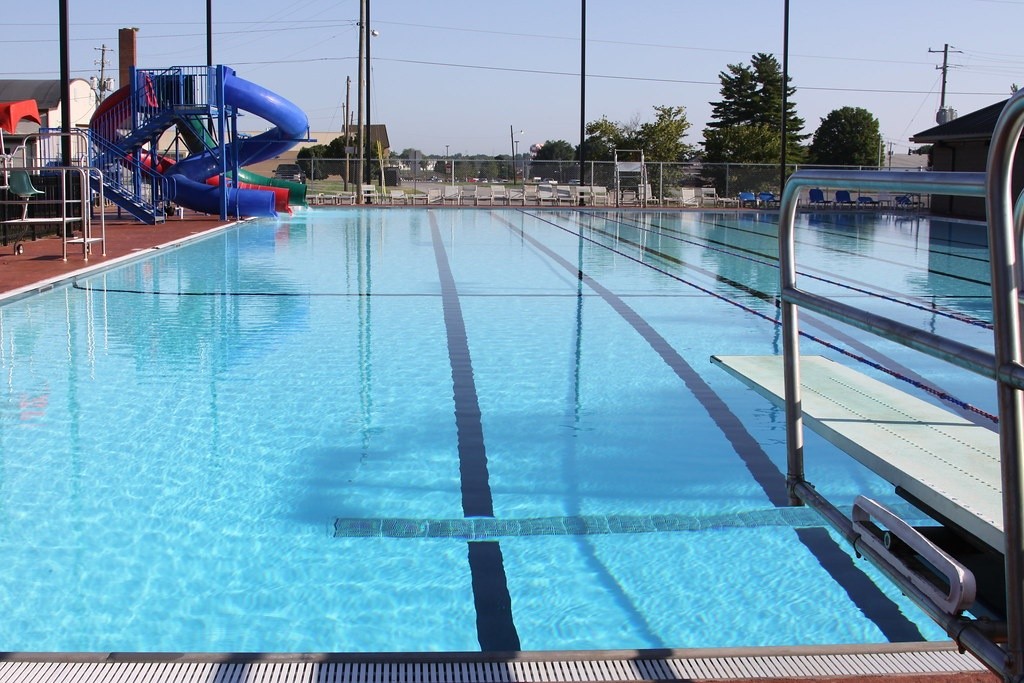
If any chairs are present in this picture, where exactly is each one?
[856,196,879,210]
[878,192,893,208]
[833,190,856,210]
[809,188,833,209]
[305,184,739,207]
[9,170,46,198]
[739,192,757,208]
[895,196,925,209]
[759,192,780,209]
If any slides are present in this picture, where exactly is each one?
[88,63,307,217]
